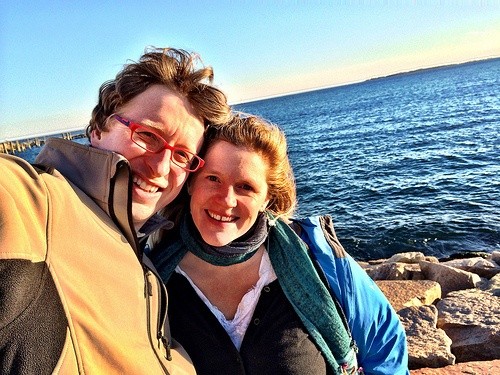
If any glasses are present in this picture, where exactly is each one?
[112,113,203,173]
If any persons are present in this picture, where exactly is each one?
[0,46,204,374]
[147,112,409,375]
